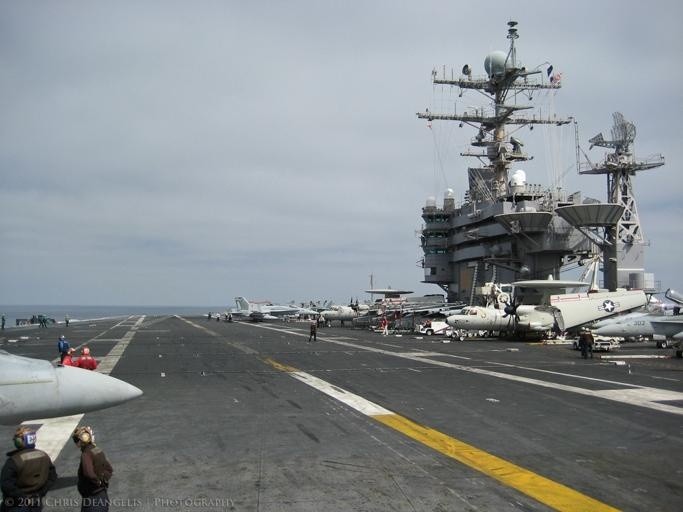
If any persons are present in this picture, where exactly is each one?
[2,311,97,371]
[578,327,594,359]
[72,426,113,512]
[208,311,233,323]
[0,426,58,512]
[381,318,389,336]
[309,314,326,342]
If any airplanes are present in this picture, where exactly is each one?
[229,296,319,322]
[0,346,145,426]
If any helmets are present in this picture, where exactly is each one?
[14,425,37,450]
[73,426,95,447]
[59,334,90,356]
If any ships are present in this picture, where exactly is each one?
[0,19,682,511]
[0,313,683,512]
